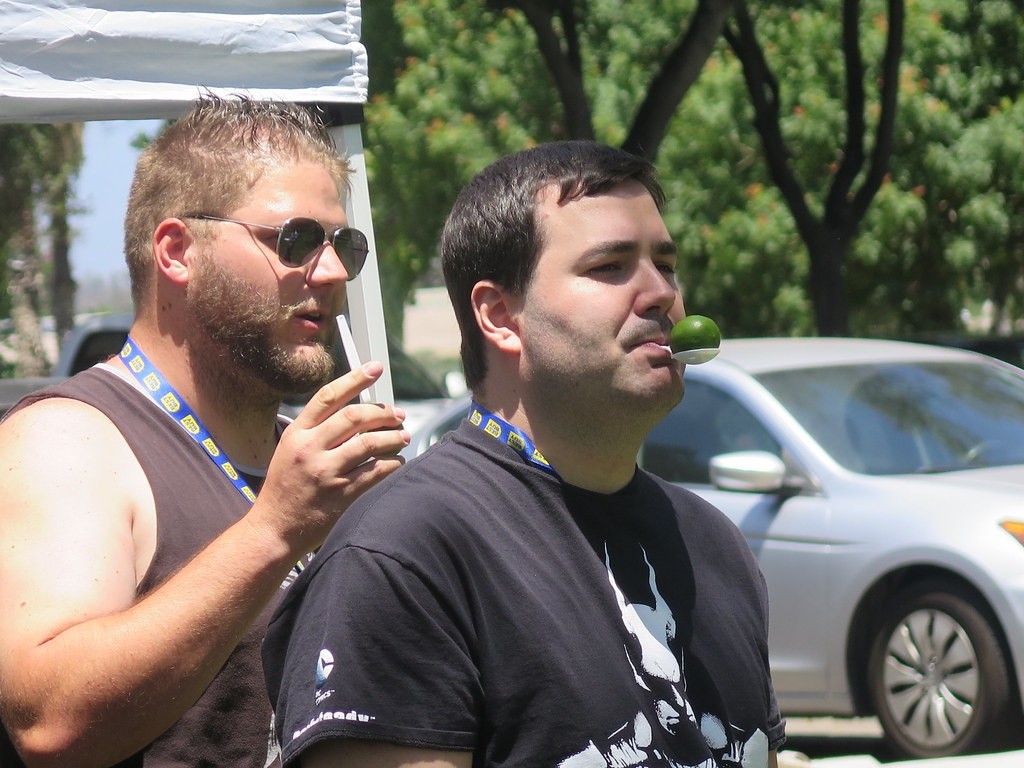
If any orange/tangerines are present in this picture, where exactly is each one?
[669,314,721,356]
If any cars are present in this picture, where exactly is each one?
[410,335,1023,757]
[59,309,373,424]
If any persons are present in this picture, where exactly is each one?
[0,95,408,768]
[261,140,786,768]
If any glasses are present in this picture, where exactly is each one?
[176,213,370,282]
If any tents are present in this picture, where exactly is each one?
[0,1,396,425]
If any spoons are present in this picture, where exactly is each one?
[661,345,720,365]
